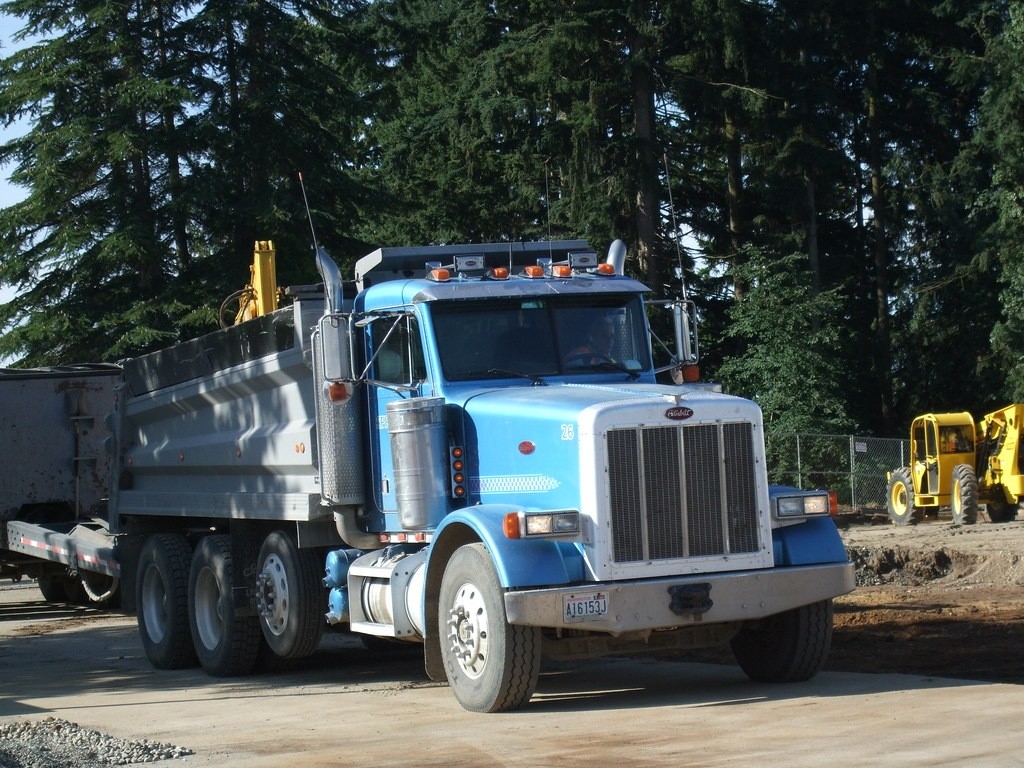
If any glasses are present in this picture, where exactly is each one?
[597,334,616,338]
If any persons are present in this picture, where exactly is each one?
[562,315,618,370]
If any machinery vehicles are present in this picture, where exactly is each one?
[882,400,1024,526]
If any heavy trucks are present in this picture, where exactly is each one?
[0,230,857,716]
